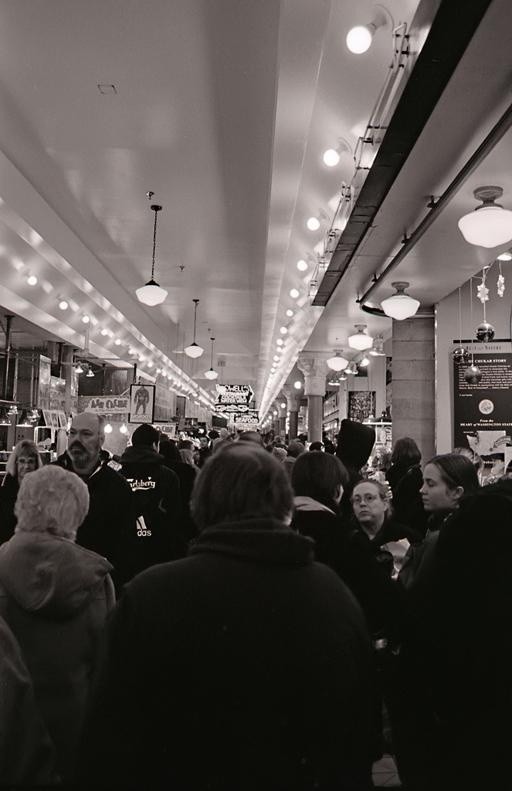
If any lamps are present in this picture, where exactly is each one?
[345,15,388,55]
[307,213,327,232]
[255,274,319,434]
[323,143,349,167]
[20,267,202,441]
[323,184,512,389]
[132,202,229,400]
[296,256,315,271]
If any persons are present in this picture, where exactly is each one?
[397,475,512,791]
[0,464,116,790]
[72,444,383,791]
